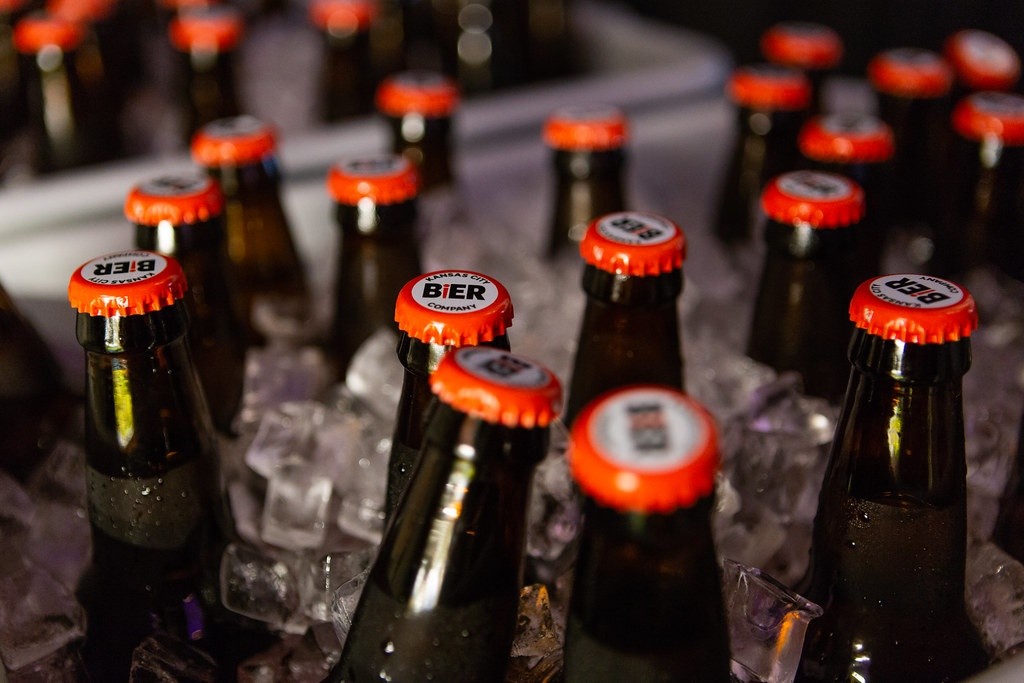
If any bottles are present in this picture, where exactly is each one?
[744,171,862,416]
[377,74,468,248]
[796,111,894,281]
[0,0,135,183]
[170,17,248,155]
[192,115,312,353]
[539,106,633,271]
[317,346,564,683]
[123,173,273,512]
[66,254,282,683]
[791,274,967,683]
[869,52,954,269]
[157,0,221,105]
[705,73,807,301]
[317,153,424,409]
[927,96,1024,330]
[720,558,823,683]
[305,0,392,127]
[945,33,1019,117]
[562,390,726,683]
[559,211,689,457]
[764,29,842,118]
[381,272,512,538]
